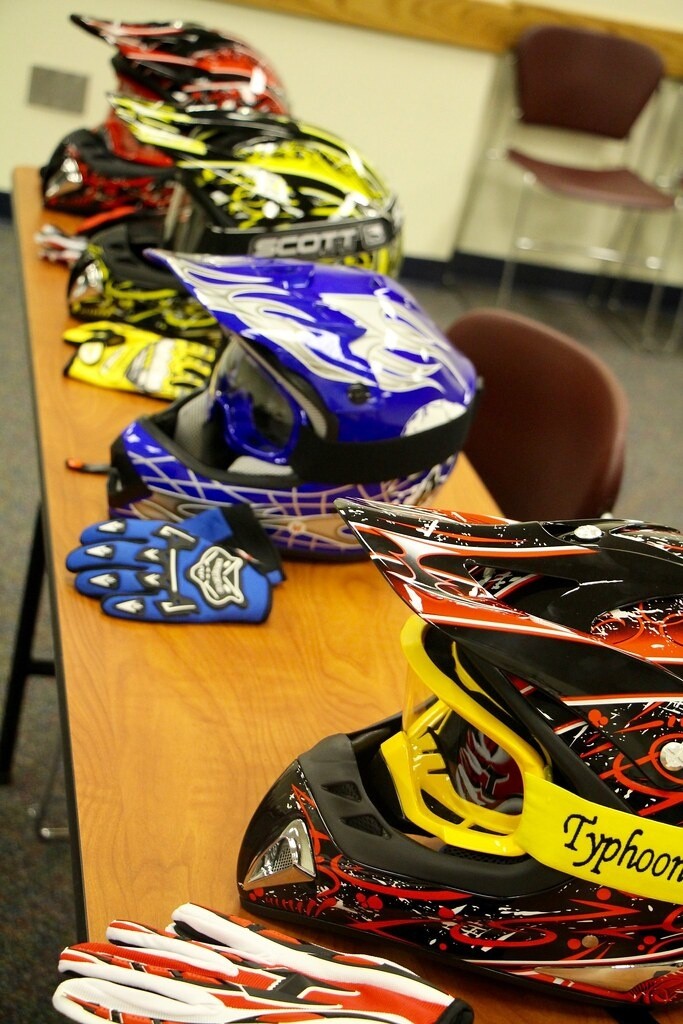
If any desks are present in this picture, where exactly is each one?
[0,169,683,1023]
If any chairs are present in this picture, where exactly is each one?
[588,72,682,352]
[440,27,674,351]
[30,305,628,846]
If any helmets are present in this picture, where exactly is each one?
[237,496,683,1014]
[40,14,290,218]
[107,248,483,564]
[68,90,402,348]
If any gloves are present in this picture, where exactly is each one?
[61,320,216,400]
[65,505,287,625]
[35,225,88,271]
[52,902,475,1024]
